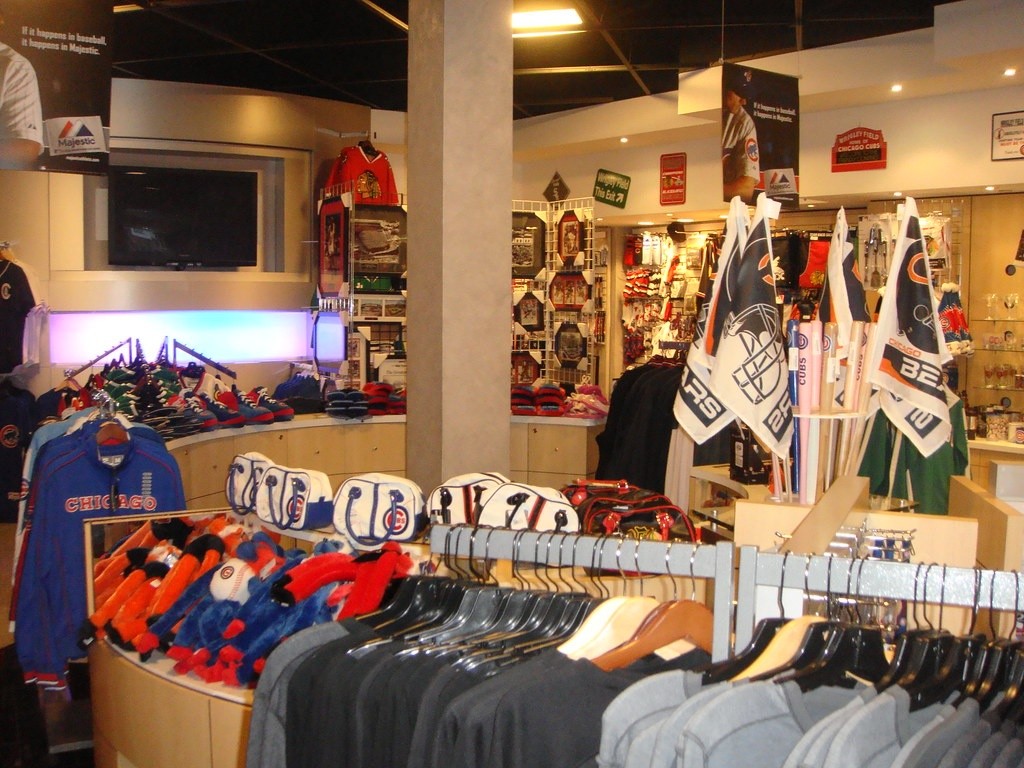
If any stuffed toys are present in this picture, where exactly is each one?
[87,515,418,700]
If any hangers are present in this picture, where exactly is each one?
[0,389,1023,768]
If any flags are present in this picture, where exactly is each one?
[673,192,970,517]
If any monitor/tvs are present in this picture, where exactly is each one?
[107,164,257,266]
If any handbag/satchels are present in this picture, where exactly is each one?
[559,477,703,577]
[477,481,582,531]
[331,472,427,551]
[225,451,274,516]
[255,465,336,532]
[427,471,511,526]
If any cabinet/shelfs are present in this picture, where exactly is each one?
[969,320,1024,390]
[353,192,609,388]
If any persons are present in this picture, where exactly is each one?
[722,61,762,203]
[0,0,45,172]
